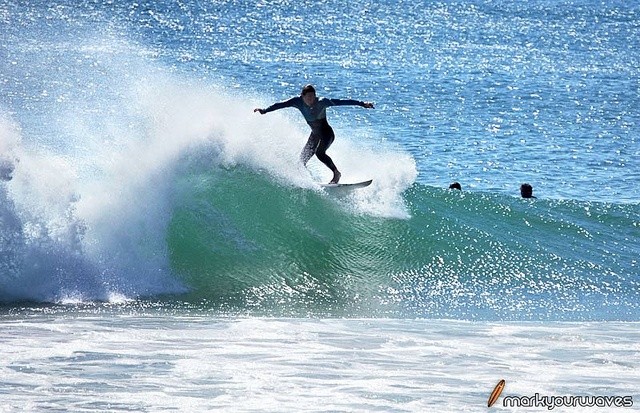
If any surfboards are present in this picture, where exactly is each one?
[319,179,372,192]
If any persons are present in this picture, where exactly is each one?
[254,83,375,184]
[448,181,462,192]
[519,183,537,199]
